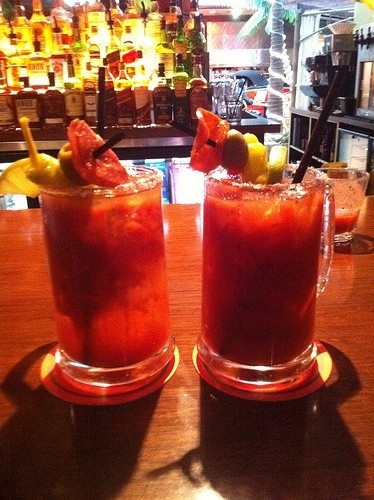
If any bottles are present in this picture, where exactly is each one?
[172,53,191,124]
[114,62,134,128]
[185,55,210,123]
[131,63,152,128]
[0,50,16,131]
[151,63,174,125]
[0,0,211,96]
[81,62,101,128]
[42,71,65,129]
[61,55,84,125]
[102,68,118,129]
[15,77,41,129]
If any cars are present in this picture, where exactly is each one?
[231,71,290,117]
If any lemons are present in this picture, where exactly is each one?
[265,146,289,186]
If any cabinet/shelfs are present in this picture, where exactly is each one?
[287,107,374,196]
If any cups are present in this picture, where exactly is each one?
[197,168,336,401]
[38,164,176,397]
[224,94,243,125]
[324,168,370,247]
[211,79,244,118]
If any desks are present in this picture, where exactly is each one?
[0,196,374,500]
[0,110,281,210]
